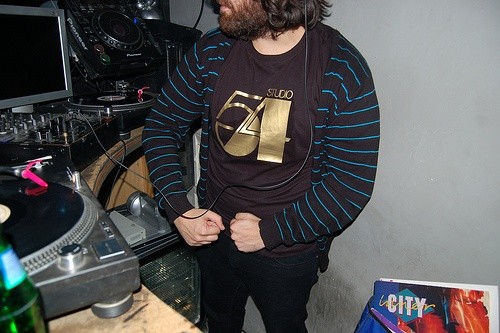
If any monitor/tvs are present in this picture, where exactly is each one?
[0,4,73,110]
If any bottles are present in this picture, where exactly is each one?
[0,222,50,333]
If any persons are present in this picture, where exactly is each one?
[141,0,381,333]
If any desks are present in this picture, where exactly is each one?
[49,127,204,333]
